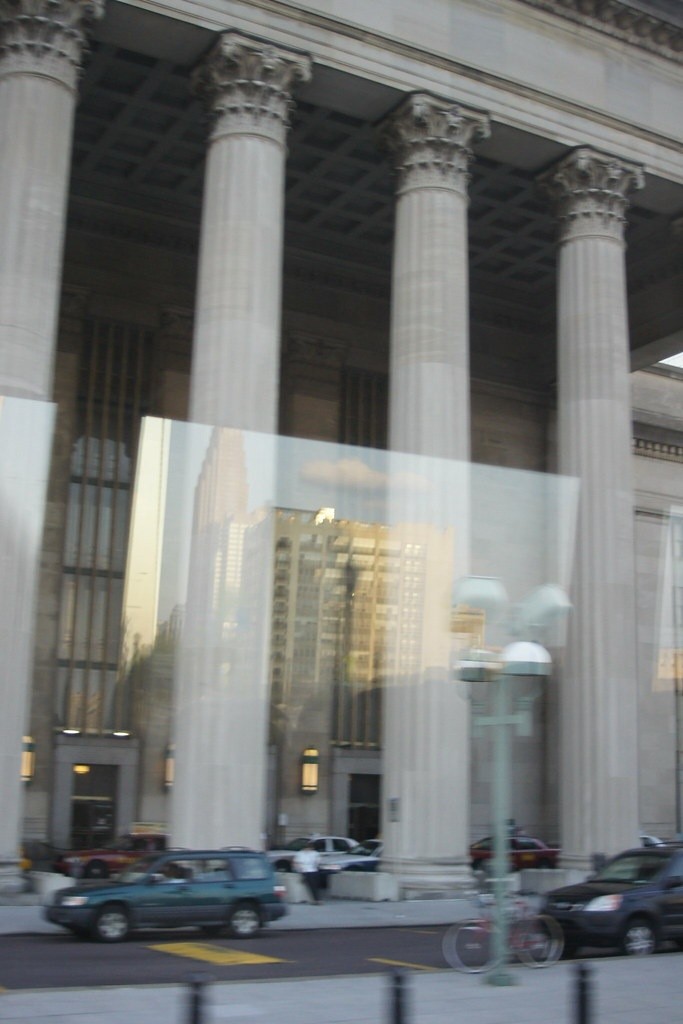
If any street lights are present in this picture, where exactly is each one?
[447,575,571,989]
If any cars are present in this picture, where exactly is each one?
[55,820,174,878]
[466,832,562,871]
[321,839,383,878]
[265,834,360,872]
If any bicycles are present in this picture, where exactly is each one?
[443,891,563,975]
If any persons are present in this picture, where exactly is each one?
[293,844,322,905]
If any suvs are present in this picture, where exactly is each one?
[41,844,291,939]
[540,838,682,957]
